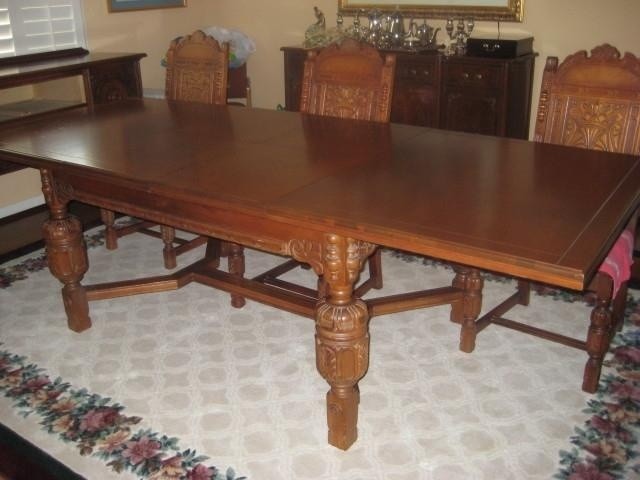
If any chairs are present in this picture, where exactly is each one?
[227,38,399,308]
[96,29,229,268]
[457,43,640,393]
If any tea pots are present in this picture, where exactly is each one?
[303,5,442,51]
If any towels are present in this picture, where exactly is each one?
[596,226,634,300]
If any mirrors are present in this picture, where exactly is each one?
[335,0,525,23]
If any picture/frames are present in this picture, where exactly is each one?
[106,0,186,14]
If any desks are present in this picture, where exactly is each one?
[0,93,640,450]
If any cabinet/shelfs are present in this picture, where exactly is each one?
[227,62,251,108]
[0,48,147,265]
[280,44,539,140]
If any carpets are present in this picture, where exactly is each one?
[0,212,639,480]
